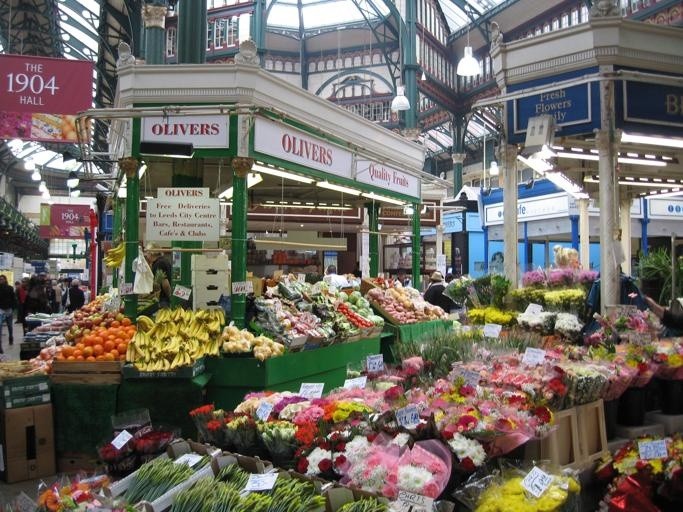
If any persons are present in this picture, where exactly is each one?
[628,291,683,415]
[582,261,645,338]
[0,269,85,356]
[393,271,408,285]
[351,262,362,278]
[322,264,337,276]
[444,273,454,282]
[552,244,579,268]
[422,271,450,316]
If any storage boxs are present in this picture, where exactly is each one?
[0,403,55,484]
[190,254,228,271]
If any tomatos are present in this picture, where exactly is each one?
[333,302,374,330]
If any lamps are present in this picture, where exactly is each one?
[455,0,482,77]
[390,0,410,111]
[61,151,118,197]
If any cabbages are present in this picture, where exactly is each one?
[338,290,385,327]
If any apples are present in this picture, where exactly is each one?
[64,304,125,339]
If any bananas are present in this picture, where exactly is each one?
[101,242,124,269]
[126,305,226,372]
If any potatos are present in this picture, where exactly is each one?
[368,286,445,324]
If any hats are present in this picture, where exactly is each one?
[429,271,444,281]
